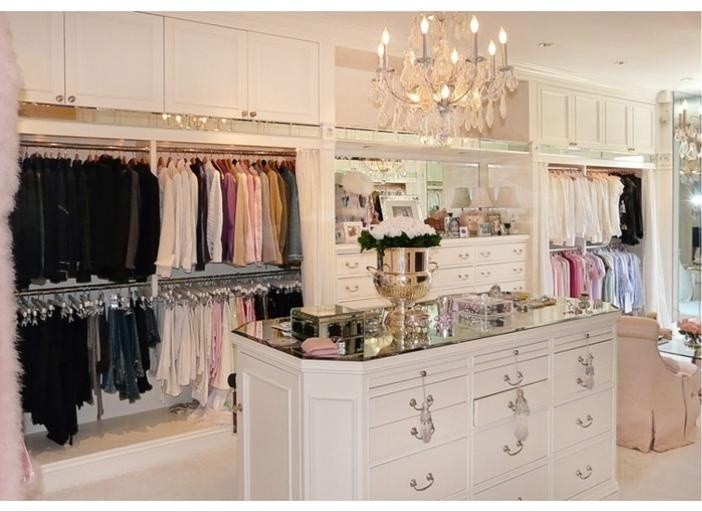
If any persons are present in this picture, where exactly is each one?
[448,218,461,239]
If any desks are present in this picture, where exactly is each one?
[657,336,701,360]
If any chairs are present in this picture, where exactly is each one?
[615,316,701,455]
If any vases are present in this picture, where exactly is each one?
[681,335,702,348]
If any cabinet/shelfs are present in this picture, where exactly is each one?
[0,11,659,501]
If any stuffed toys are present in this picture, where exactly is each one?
[460,209,487,233]
[424,207,452,236]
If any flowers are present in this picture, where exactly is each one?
[676,318,702,334]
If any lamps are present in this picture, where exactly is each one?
[674,96,702,213]
